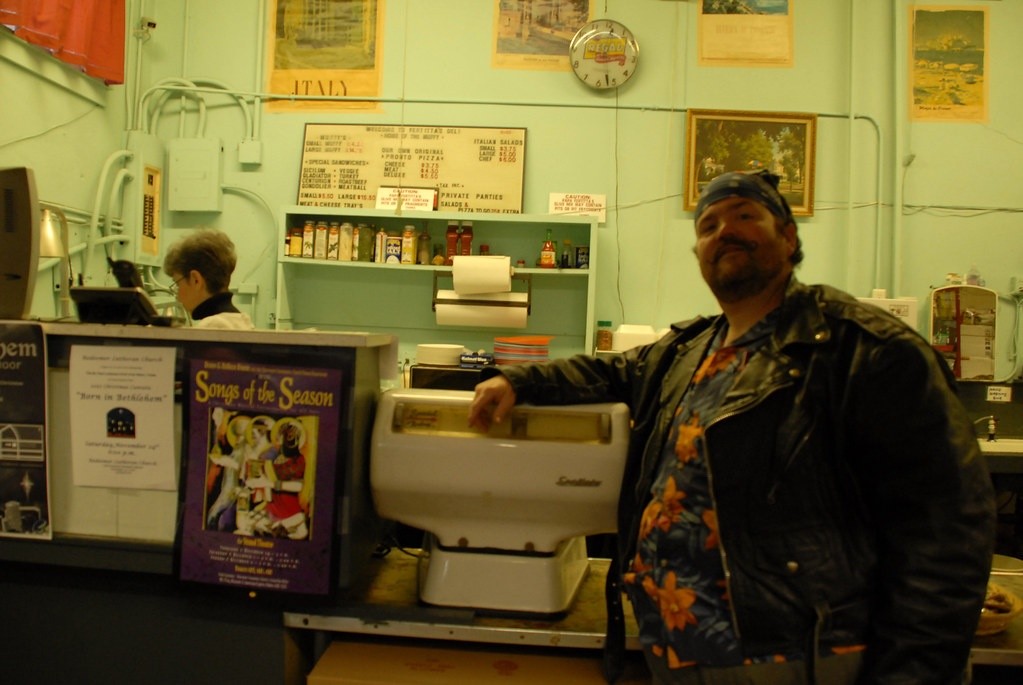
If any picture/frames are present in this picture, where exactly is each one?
[683,108,817,216]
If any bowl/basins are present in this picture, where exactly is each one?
[973,554,1023,636]
[613,325,672,353]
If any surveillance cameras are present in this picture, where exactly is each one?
[141,16,157,29]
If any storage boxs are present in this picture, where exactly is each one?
[308,642,607,685]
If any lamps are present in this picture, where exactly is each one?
[40,208,70,317]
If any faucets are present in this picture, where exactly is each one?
[973,416,999,442]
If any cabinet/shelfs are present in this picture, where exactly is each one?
[275,204,599,371]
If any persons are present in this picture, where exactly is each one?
[164,231,254,328]
[467,169,996,685]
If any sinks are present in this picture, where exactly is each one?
[976,439,1023,453]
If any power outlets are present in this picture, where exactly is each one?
[402,350,416,368]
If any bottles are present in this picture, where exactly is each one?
[283,215,573,269]
[597,320,612,350]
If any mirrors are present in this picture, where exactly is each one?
[929,286,999,382]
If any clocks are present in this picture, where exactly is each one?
[568,19,640,90]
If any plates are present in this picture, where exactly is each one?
[414,336,559,366]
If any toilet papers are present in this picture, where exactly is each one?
[436,255,528,328]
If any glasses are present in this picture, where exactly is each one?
[168,274,189,295]
